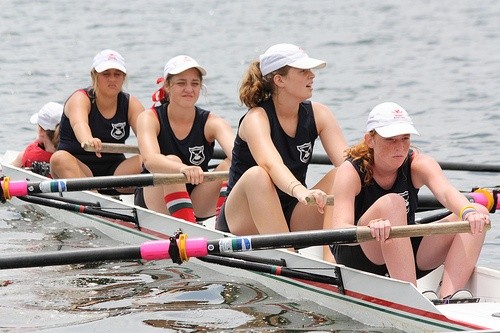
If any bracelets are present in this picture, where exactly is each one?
[288,181,301,197]
[460,205,479,221]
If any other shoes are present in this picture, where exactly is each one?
[423,290,439,300]
[444,288,473,300]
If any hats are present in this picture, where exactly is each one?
[93,49,128,74]
[164,55,206,80]
[259,43,327,77]
[30,101,64,130]
[368,102,420,139]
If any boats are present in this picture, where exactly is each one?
[1,154,500,332]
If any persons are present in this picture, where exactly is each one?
[135,55,236,224]
[329,102,490,299]
[10,101,64,179]
[216,43,350,261]
[50,49,145,195]
